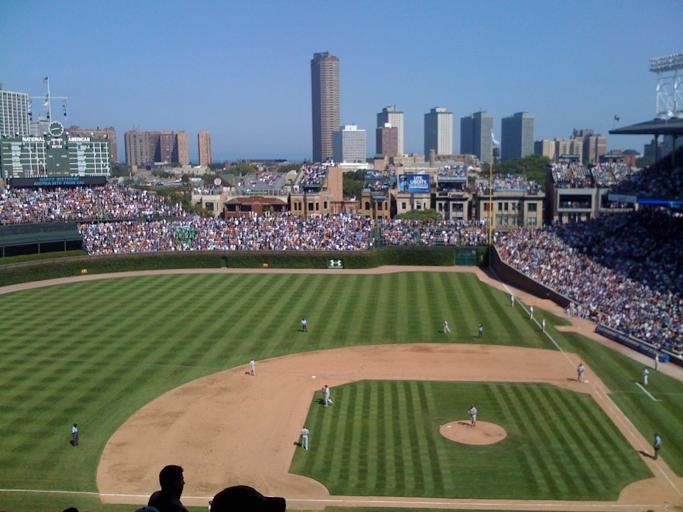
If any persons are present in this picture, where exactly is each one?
[136,465,286,512]
[300,425,310,451]
[323,385,335,407]
[467,404,477,425]
[653,432,662,460]
[72,423,79,447]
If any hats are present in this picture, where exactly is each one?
[210,485,288,512]
